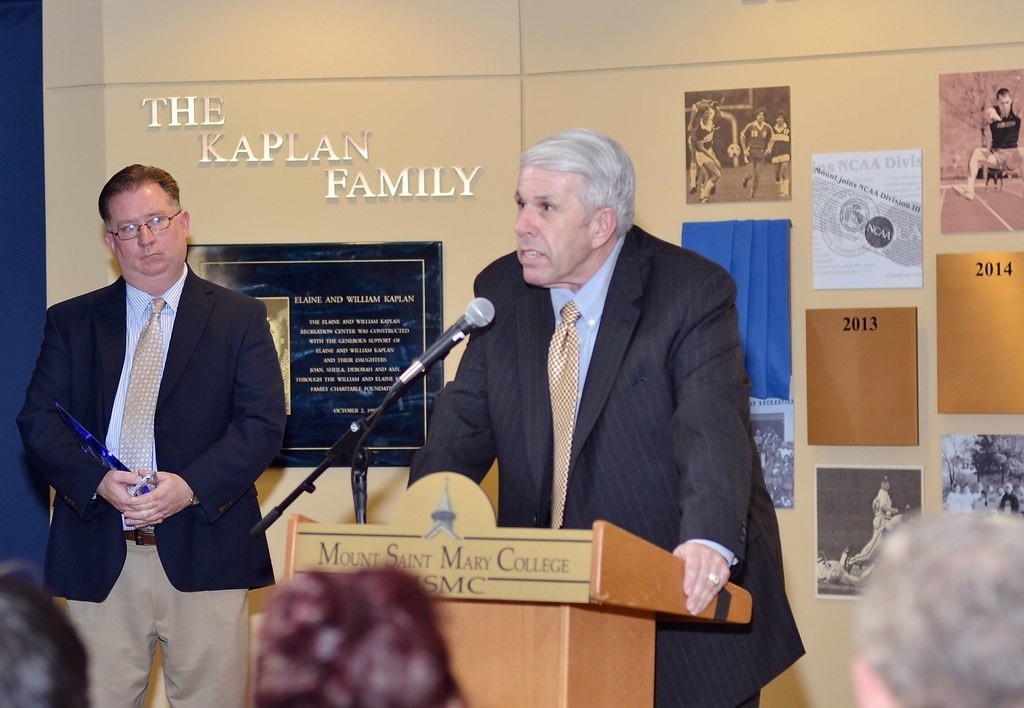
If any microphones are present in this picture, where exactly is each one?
[390,297,495,396]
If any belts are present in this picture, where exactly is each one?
[123,531,156,545]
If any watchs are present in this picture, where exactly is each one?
[189,493,200,508]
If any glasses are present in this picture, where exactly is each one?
[107,210,182,241]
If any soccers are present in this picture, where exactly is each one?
[727,143,741,158]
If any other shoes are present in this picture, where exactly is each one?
[689,179,717,204]
[780,192,789,199]
[751,189,755,197]
[846,557,853,574]
[743,176,748,189]
[952,184,974,201]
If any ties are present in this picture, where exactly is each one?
[548,301,582,529]
[118,298,169,529]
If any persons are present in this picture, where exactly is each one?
[952,88,1023,202]
[841,475,899,569]
[0,570,88,708]
[257,560,465,707]
[16,162,286,708]
[752,426,793,506]
[851,505,1024,708]
[686,92,791,203]
[945,482,1024,516]
[406,127,806,708]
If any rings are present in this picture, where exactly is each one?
[710,573,721,586]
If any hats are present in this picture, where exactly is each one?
[880,475,889,483]
